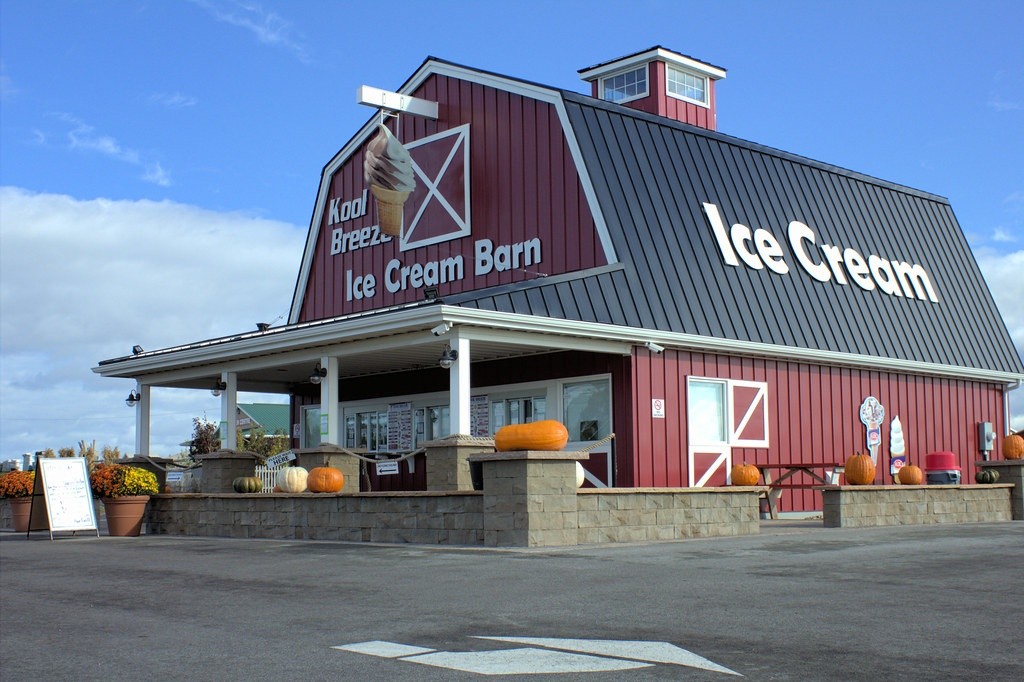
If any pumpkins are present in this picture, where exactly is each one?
[898,462,922,485]
[731,461,760,486]
[974,469,999,484]
[276,465,310,493]
[845,451,876,485]
[233,476,263,493]
[493,420,568,451]
[1002,432,1024,460]
[307,461,345,493]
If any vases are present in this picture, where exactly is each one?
[8,497,43,532]
[102,494,151,536]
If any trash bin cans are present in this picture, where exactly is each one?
[926,470,960,485]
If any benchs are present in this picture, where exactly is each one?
[720,483,837,520]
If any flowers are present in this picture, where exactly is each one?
[0,470,36,498]
[90,463,159,499]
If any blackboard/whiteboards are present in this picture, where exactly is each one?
[29,457,97,530]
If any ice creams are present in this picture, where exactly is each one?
[363,123,415,237]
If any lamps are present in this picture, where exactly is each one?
[424,287,438,299]
[256,323,270,331]
[439,344,459,368]
[308,363,327,384]
[211,377,227,396]
[124,390,140,407]
[132,346,144,355]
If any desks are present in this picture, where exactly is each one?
[753,462,847,519]
